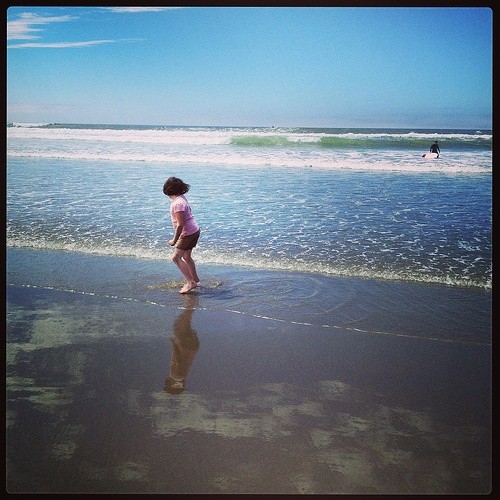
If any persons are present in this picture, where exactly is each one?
[429,141,440,154]
[164,294,199,394]
[163,177,201,293]
